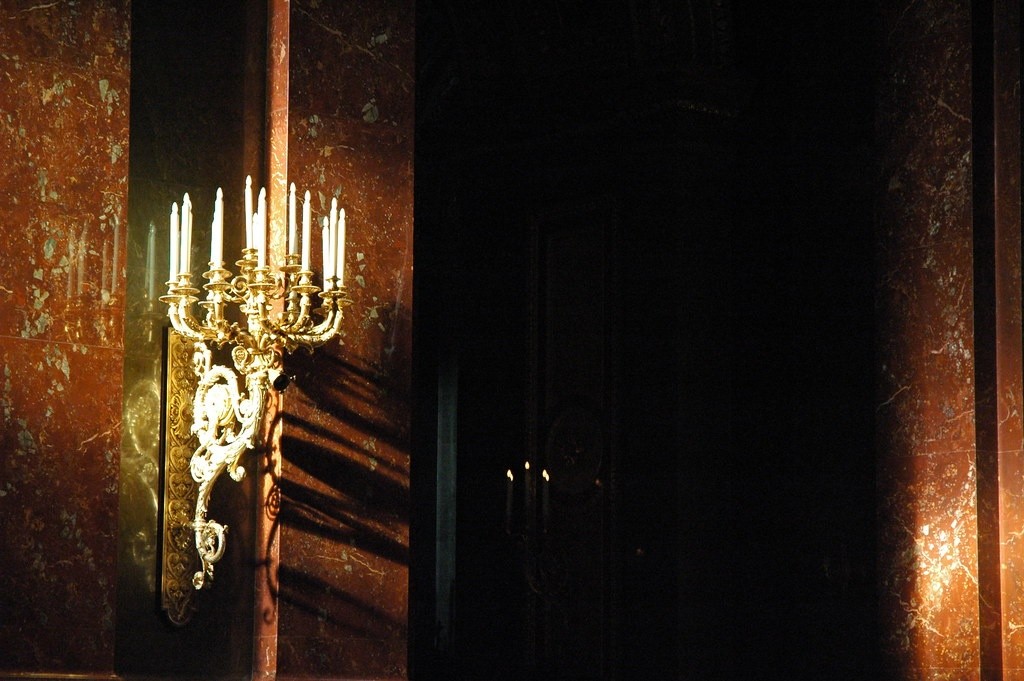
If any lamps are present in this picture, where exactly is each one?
[169,175,349,591]
